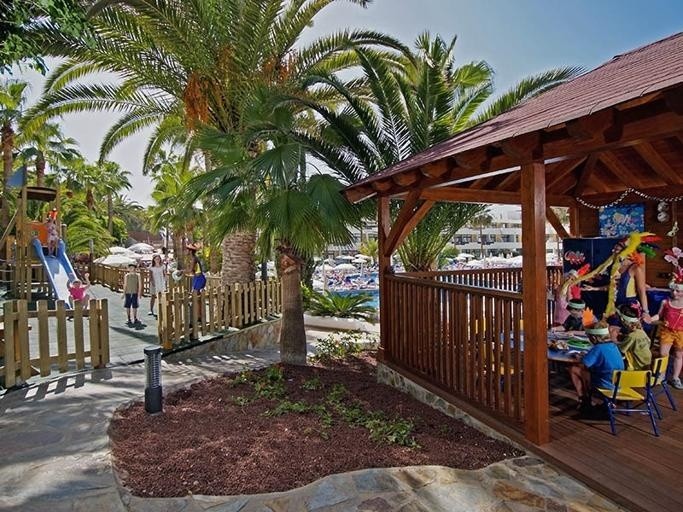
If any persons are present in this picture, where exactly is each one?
[66,272,90,309]
[443,237,563,271]
[45,208,60,259]
[149,255,167,315]
[553,242,683,409]
[311,261,404,291]
[120,264,142,324]
[176,243,206,322]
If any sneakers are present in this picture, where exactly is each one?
[669,377,683,389]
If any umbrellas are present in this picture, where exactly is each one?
[255,271,275,280]
[258,260,275,272]
[313,253,371,263]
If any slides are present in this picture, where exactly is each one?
[34,238,95,309]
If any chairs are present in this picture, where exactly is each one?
[467,320,677,437]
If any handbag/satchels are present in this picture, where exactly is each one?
[192,273,206,290]
